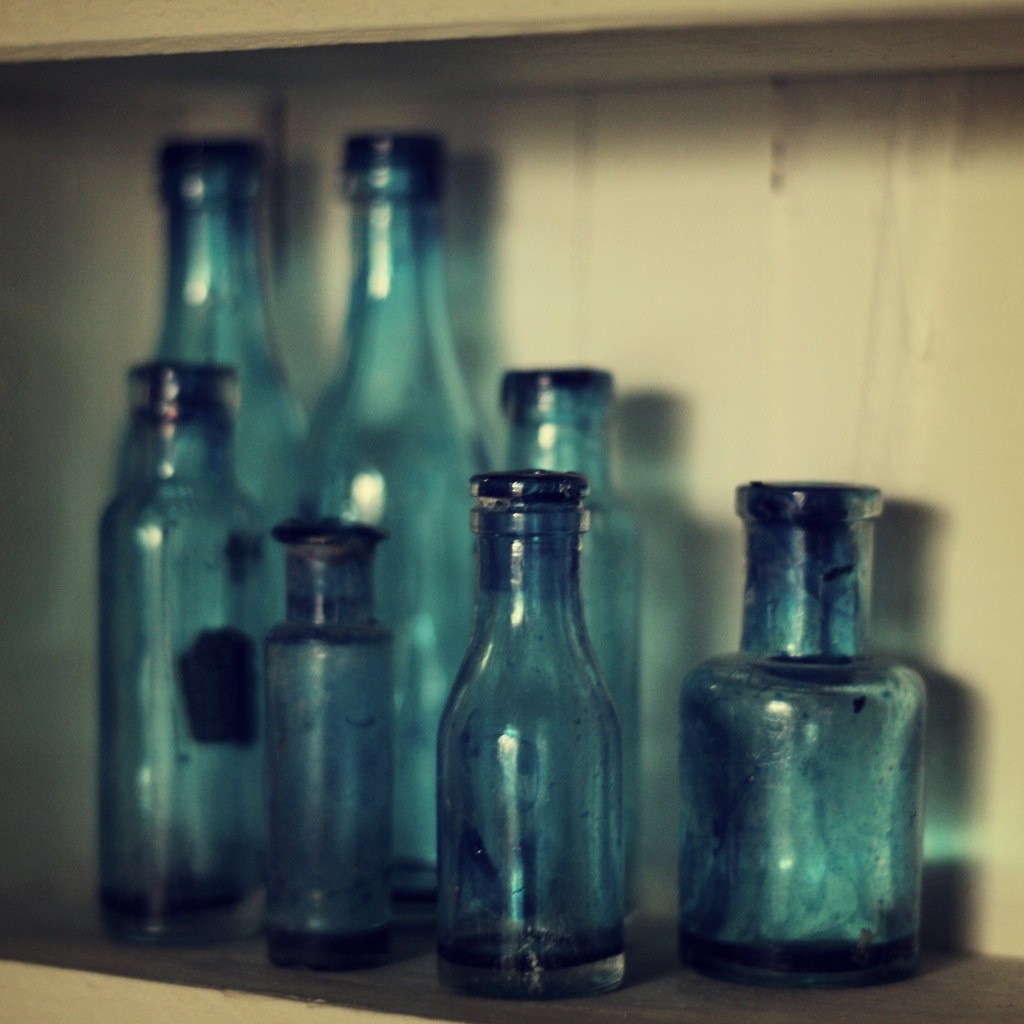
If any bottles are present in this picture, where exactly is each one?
[158,131,316,630]
[677,481,927,990]
[310,131,501,914]
[503,363,639,916]
[260,521,384,966]
[436,473,623,1000]
[100,359,264,924]
